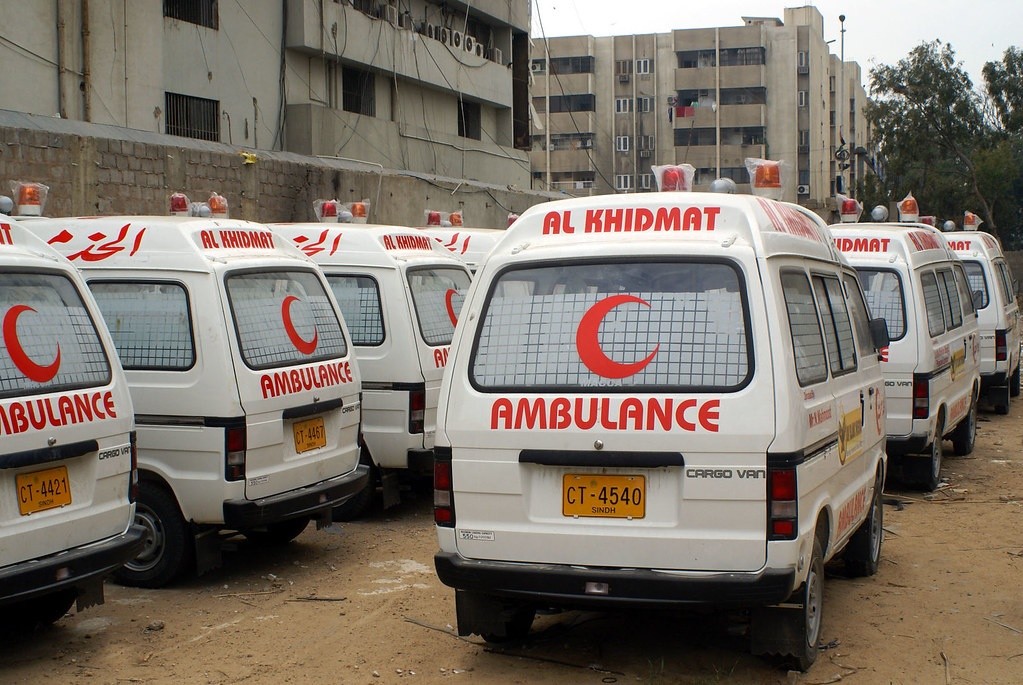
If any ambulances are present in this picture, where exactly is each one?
[827,191,982,491]
[0,180,370,589]
[919,210,1021,415]
[311,211,507,278]
[434,157,890,672]
[170,191,474,522]
[0,213,147,627]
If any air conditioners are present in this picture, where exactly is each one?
[531,64,542,73]
[797,184,809,195]
[335,0,503,67]
[799,144,809,154]
[798,66,810,74]
[641,151,651,158]
[619,74,629,82]
